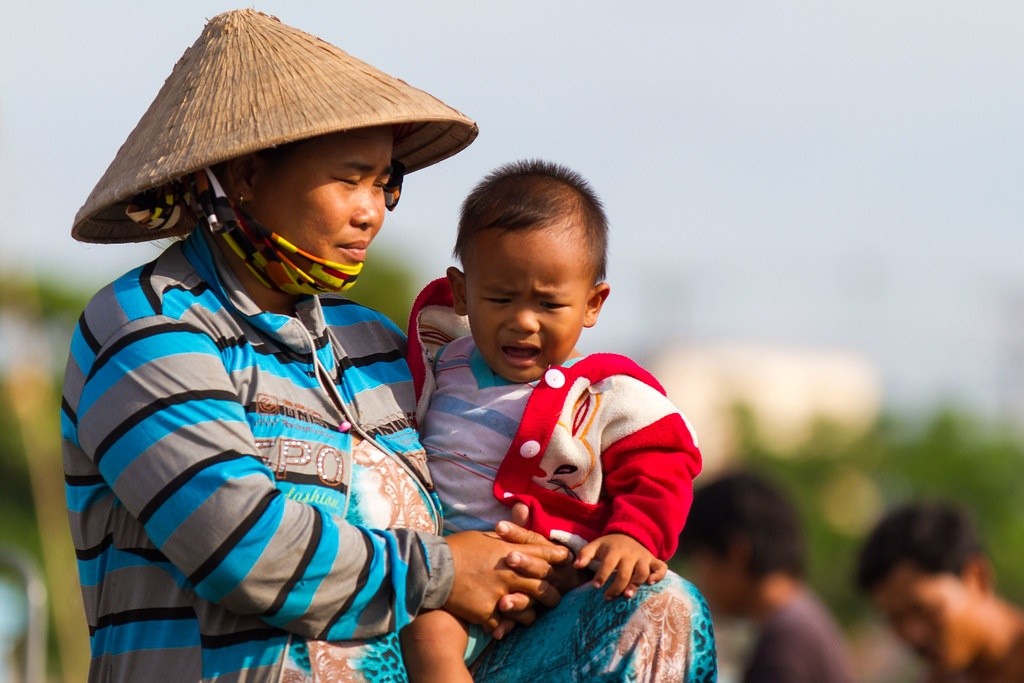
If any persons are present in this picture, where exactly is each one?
[676,470,1024,683]
[60,8,713,683]
[406,160,702,683]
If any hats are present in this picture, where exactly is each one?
[70,10,479,243]
